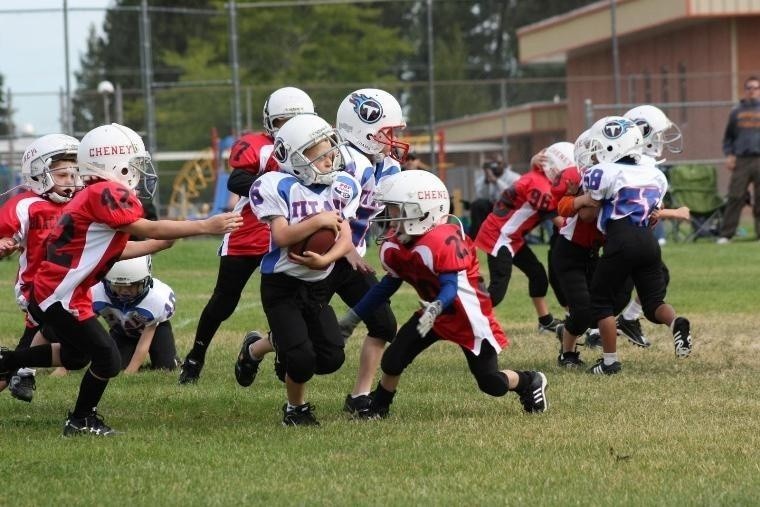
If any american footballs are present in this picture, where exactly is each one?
[288,213,336,260]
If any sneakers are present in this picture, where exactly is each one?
[10,368,36,402]
[0,346,12,391]
[62,407,122,436]
[537,313,691,375]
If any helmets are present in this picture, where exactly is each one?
[263,87,450,241]
[542,105,683,184]
[22,123,159,308]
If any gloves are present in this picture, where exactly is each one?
[417,300,442,337]
[338,310,360,336]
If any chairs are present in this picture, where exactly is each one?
[668,162,727,243]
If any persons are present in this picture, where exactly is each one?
[0,132,82,402]
[48,254,180,376]
[354,169,549,419]
[0,122,243,439]
[467,105,692,374]
[179,86,428,427]
[715,77,760,245]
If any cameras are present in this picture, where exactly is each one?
[484,162,502,177]
